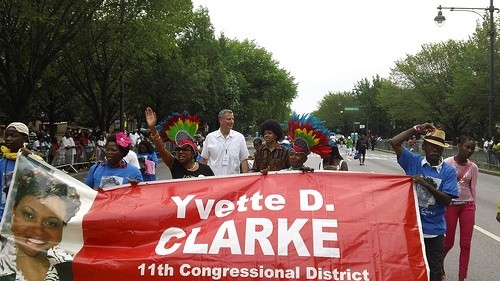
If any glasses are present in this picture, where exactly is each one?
[289,151,300,157]
[176,147,193,153]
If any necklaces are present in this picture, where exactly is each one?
[187,162,196,170]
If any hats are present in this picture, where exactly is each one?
[287,112,335,157]
[420,130,451,148]
[163,115,198,155]
[7,121,31,143]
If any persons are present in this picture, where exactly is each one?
[318,141,349,171]
[249,138,262,160]
[331,131,382,165]
[84,132,144,191]
[0,171,82,281]
[195,131,206,162]
[145,107,215,179]
[200,110,251,176]
[390,123,459,281]
[282,137,294,150]
[261,147,314,175]
[252,120,291,171]
[484,136,500,168]
[442,134,478,281]
[123,128,158,181]
[0,122,44,222]
[29,124,109,173]
[496,200,500,222]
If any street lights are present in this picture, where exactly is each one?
[434,0,500,165]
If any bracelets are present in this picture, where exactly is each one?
[414,125,421,132]
[151,131,160,140]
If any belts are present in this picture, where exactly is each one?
[450,201,467,205]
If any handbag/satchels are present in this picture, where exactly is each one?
[354,150,360,159]
